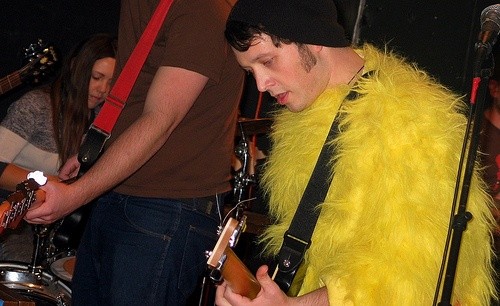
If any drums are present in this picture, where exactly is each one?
[51,256,77,288]
[0,262,74,306]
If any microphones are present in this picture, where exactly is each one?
[474,4,500,57]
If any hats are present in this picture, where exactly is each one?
[228,0,351,47]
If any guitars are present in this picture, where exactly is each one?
[0,174,82,239]
[201,217,329,306]
[0,38,58,96]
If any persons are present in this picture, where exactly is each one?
[215,0,500,306]
[476,69,500,234]
[22,0,245,306]
[1,32,117,267]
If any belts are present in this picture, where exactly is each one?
[180,197,218,217]
